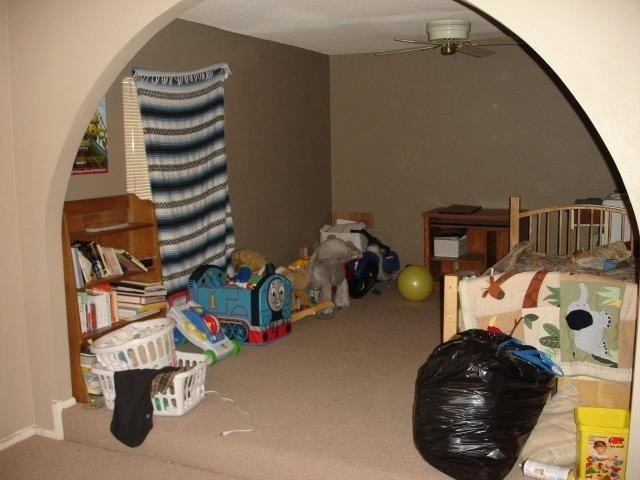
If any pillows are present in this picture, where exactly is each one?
[515,378,590,471]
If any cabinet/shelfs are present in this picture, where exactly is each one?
[58,192,179,406]
[420,203,523,296]
[570,196,634,248]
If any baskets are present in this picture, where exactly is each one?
[92,349,211,418]
[87,317,179,372]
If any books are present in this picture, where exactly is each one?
[71,241,168,336]
[85,220,129,232]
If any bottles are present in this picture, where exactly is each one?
[518,458,577,480]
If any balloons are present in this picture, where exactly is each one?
[397,265,433,302]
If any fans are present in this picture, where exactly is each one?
[369,19,520,60]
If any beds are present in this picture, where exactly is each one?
[437,195,638,421]
